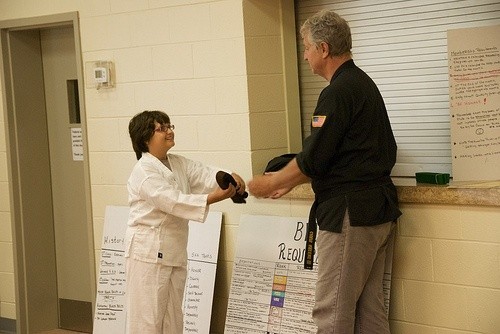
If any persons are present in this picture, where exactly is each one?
[248,10,403,334]
[125,110,245,334]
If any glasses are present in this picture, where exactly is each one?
[153,123,176,134]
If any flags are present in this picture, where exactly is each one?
[311,116,326,127]
